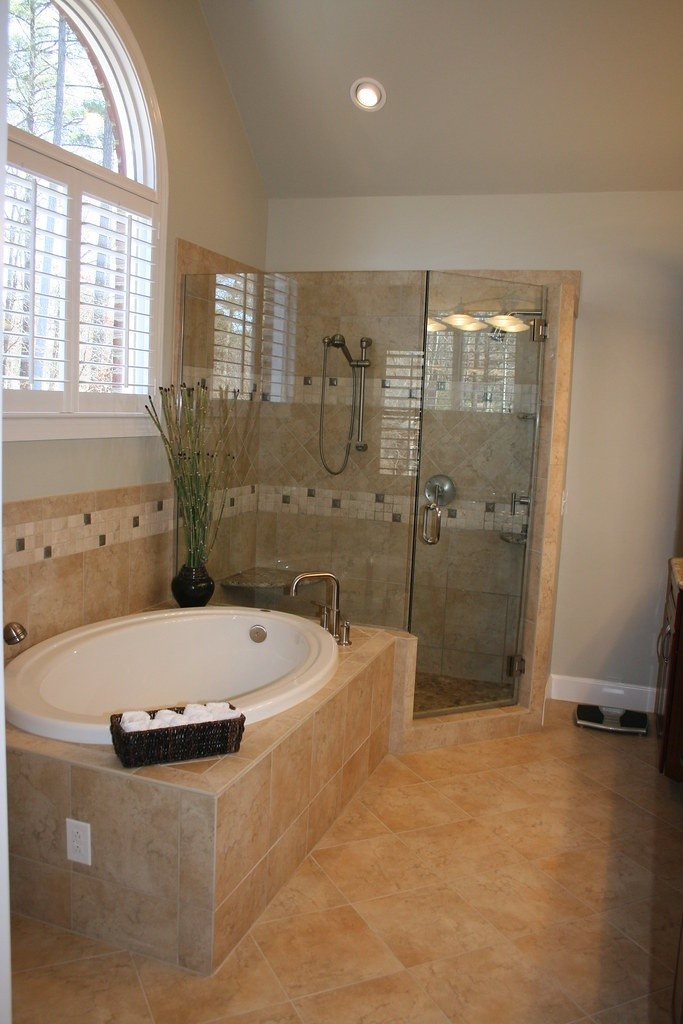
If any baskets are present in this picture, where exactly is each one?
[110,701,246,769]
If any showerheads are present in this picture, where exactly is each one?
[489,310,513,344]
[331,334,355,368]
[3,621,28,646]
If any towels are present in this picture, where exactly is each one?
[119,702,242,768]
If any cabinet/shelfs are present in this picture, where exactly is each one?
[655,557,683,785]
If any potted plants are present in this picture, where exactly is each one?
[144,384,239,608]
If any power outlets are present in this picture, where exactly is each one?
[66,818,92,867]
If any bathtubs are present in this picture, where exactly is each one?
[4,604,396,981]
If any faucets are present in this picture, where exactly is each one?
[290,571,341,645]
